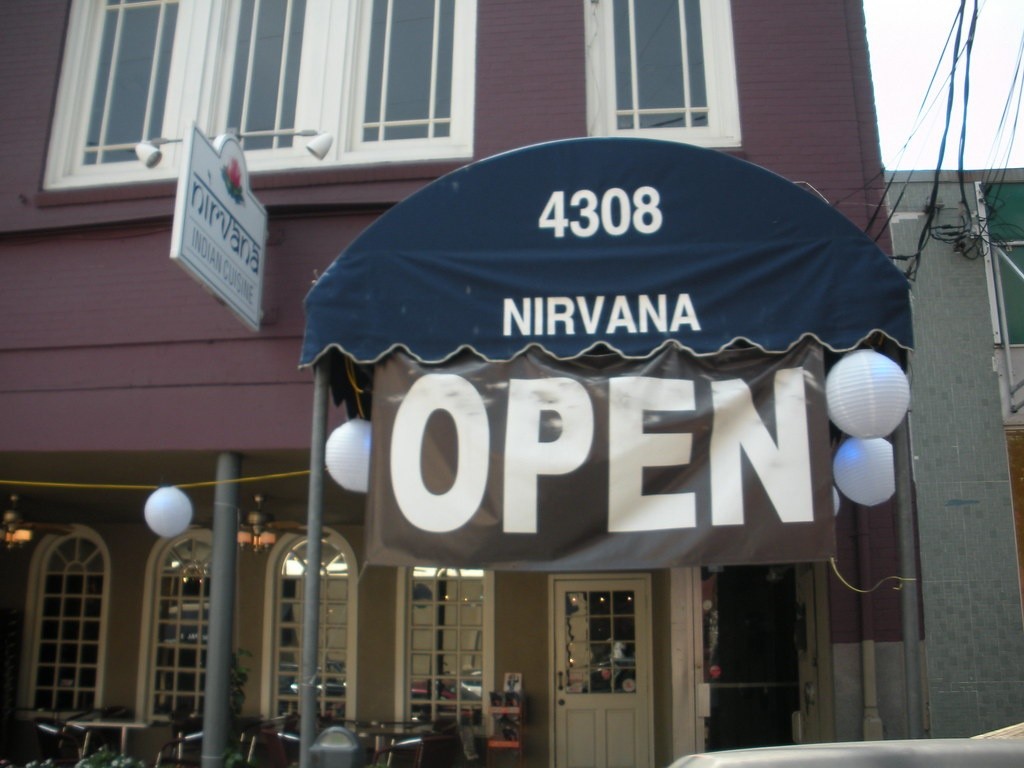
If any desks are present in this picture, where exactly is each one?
[320,719,443,763]
[66,712,154,756]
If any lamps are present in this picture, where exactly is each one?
[0,493,33,550]
[238,129,333,159]
[826,340,910,509]
[143,484,191,538]
[326,410,372,493]
[240,493,275,555]
[133,138,216,169]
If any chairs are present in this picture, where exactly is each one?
[0,707,462,768]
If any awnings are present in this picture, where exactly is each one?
[297,139,928,768]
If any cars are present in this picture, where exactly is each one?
[276,651,483,726]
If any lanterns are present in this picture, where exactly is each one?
[144,486,194,537]
[325,416,373,492]
[833,436,897,507]
[823,348,910,439]
